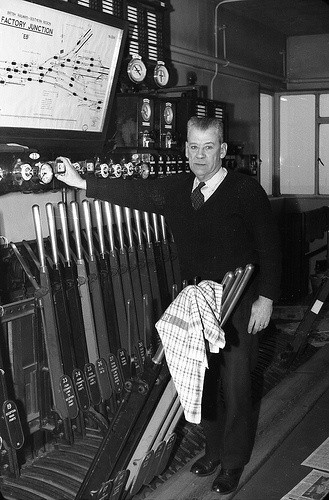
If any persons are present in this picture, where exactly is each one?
[54,115,284,495]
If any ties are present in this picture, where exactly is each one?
[190,182,206,210]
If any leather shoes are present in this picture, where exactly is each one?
[190,455,220,477]
[211,465,244,495]
